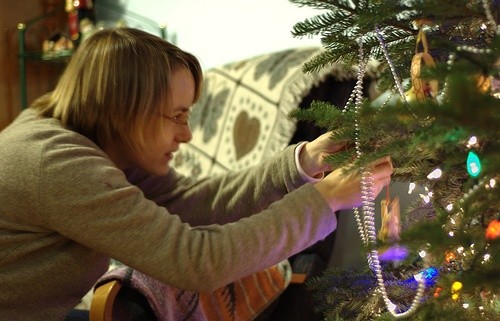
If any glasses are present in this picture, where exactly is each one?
[148,111,189,131]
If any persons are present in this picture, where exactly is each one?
[0,27,393,321]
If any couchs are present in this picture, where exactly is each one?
[62,44,372,321]
